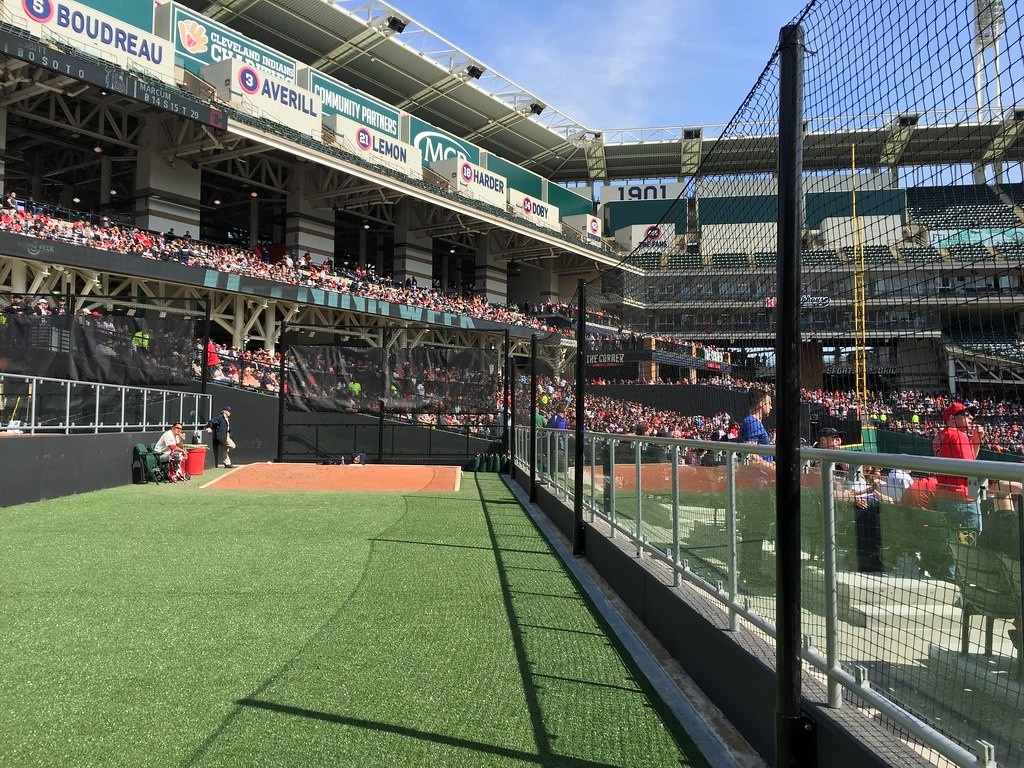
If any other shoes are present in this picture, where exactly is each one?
[225,464,233,468]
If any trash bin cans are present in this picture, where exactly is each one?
[184,444,208,475]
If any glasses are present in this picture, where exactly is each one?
[954,411,975,417]
[173,426,182,430]
[826,435,842,439]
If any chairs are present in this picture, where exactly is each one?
[135,443,172,485]
[149,443,174,483]
[946,541,1024,683]
[801,486,979,575]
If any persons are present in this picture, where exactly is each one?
[736,388,776,587]
[206,405,239,468]
[933,402,985,614]
[0,190,531,440]
[152,423,188,483]
[531,294,1023,584]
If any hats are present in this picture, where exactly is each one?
[819,428,847,437]
[943,402,977,422]
[222,406,233,410]
